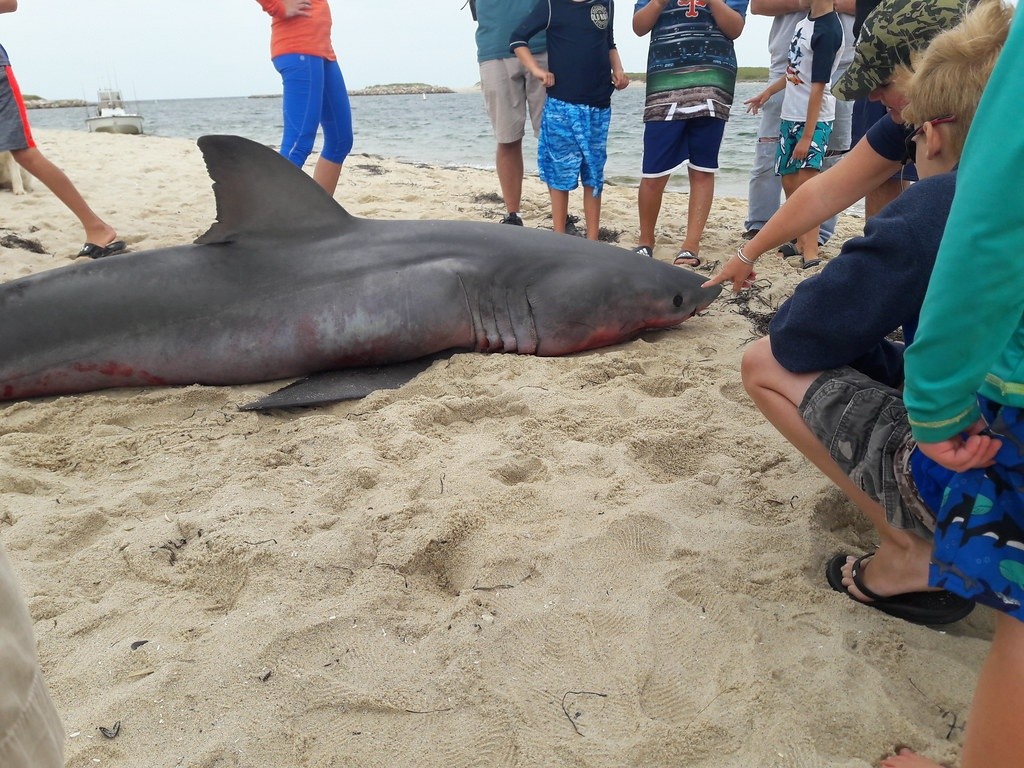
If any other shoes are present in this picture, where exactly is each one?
[800,257,821,269]
[566,214,584,238]
[498,212,523,226]
[778,241,801,260]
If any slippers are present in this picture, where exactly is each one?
[825,552,977,626]
[631,245,653,257]
[77,240,125,259]
[673,250,701,268]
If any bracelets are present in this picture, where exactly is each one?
[737,247,759,265]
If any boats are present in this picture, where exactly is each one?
[85,75,145,135]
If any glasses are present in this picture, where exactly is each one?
[905,114,956,163]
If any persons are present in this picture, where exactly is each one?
[253,0,355,197]
[0,0,126,261]
[465,3,1024,768]
